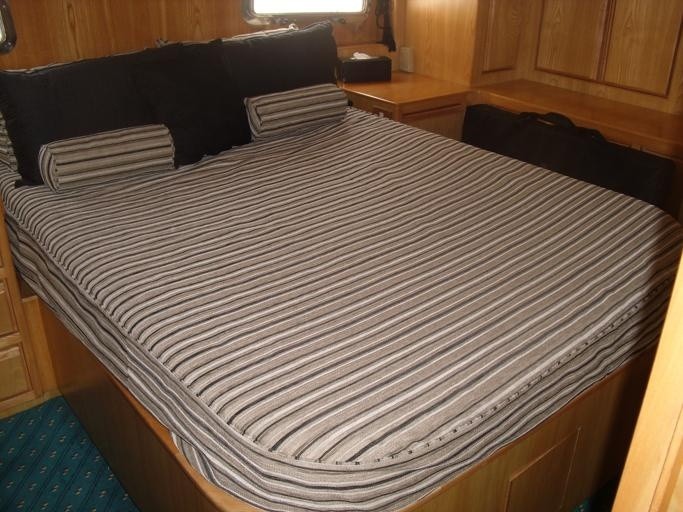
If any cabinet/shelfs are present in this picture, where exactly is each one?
[405,0,681,160]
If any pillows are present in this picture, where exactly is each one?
[41,123,176,190]
[244,83,347,143]
[2,38,251,186]
[210,23,341,143]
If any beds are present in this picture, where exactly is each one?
[0,26,679,509]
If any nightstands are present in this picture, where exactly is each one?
[0,224,49,420]
[342,71,472,153]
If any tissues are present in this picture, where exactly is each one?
[337,51,392,84]
